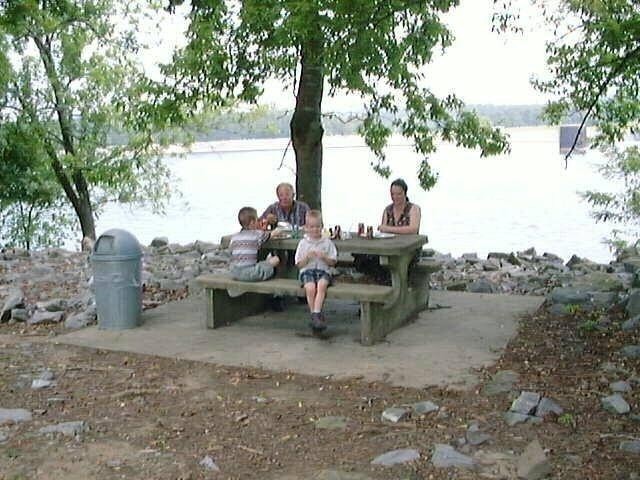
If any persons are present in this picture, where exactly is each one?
[258,183,310,312]
[294,210,337,336]
[356,180,420,288]
[226,206,280,282]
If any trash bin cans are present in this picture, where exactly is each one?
[91,228,143,329]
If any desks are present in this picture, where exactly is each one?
[206,233,429,346]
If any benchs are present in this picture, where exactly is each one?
[196,258,442,347]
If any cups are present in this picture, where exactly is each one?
[270,217,278,231]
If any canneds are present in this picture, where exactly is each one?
[334,225,341,239]
[366,226,374,239]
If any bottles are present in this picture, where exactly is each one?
[367,225,373,240]
[334,225,342,240]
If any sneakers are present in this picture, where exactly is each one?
[311,313,328,331]
[272,298,284,312]
